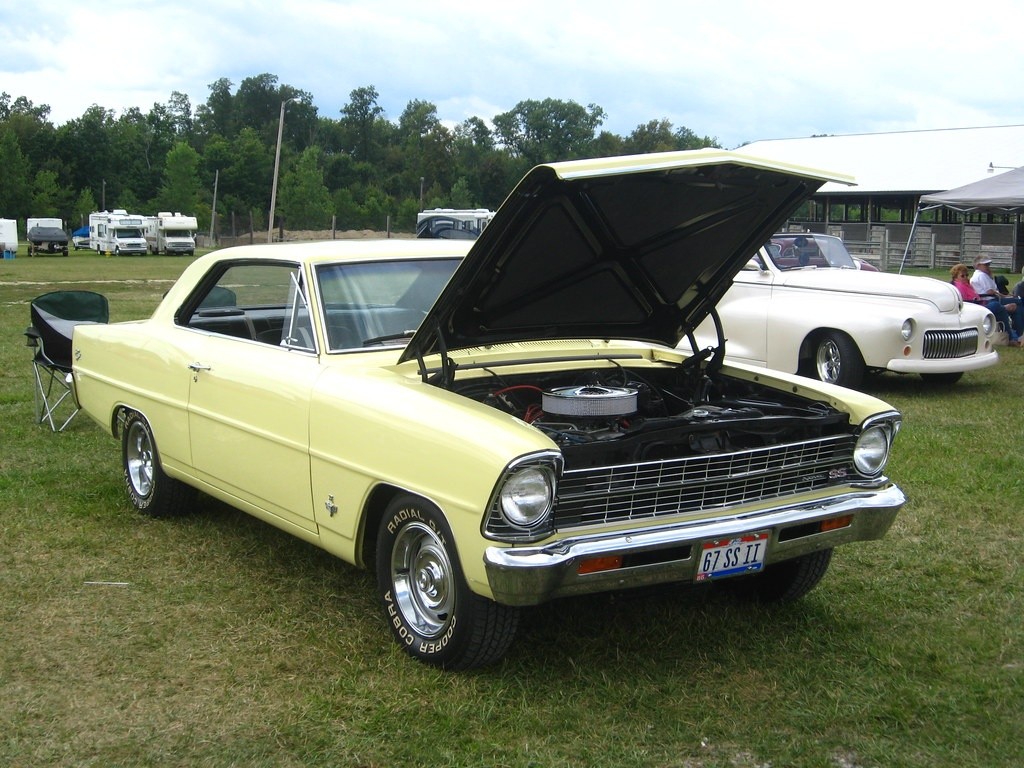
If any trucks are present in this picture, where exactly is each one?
[144,211,198,257]
[416,205,496,238]
[88,209,148,256]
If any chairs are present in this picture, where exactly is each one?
[952,280,1001,307]
[258,326,357,349]
[163,285,236,308]
[24,290,110,433]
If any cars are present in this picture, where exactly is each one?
[61,146,908,680]
[676,228,1000,392]
[762,233,878,274]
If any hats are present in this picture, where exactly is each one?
[970,253,993,266]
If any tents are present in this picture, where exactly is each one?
[899,164,1024,275]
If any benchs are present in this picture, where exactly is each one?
[190,307,427,345]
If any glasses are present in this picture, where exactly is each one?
[958,273,969,278]
[985,262,991,267]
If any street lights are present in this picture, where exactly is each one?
[266,97,304,243]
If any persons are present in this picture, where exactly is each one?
[951,253,1024,348]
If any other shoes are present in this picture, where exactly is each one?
[1009,339,1023,348]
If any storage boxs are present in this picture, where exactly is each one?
[4,250,16,259]
